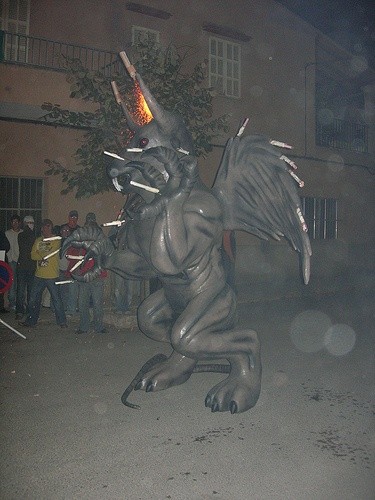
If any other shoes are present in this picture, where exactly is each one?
[57,322,67,328]
[19,321,32,326]
[15,315,23,320]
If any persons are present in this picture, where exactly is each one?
[41,210,81,306]
[75,211,109,334]
[22,218,69,328]
[0,215,38,320]
[108,227,134,315]
[222,229,240,295]
